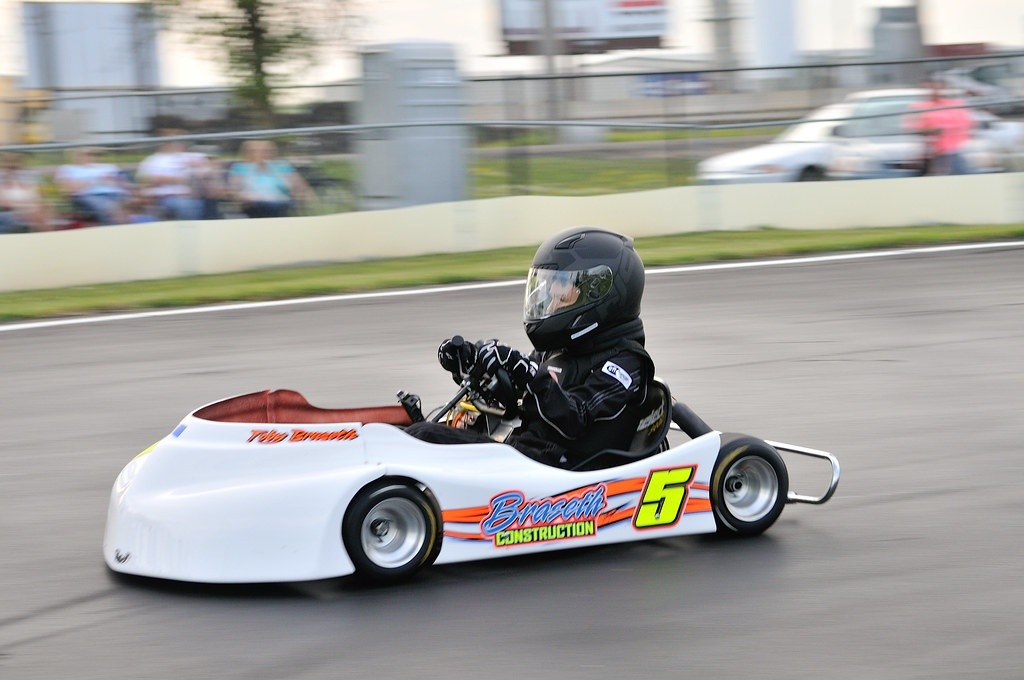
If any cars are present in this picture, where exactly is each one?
[694,89,1024,181]
[932,60,1024,115]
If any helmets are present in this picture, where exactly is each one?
[523,227,644,351]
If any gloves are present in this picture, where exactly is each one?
[437,338,475,372]
[477,344,529,383]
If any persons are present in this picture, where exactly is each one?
[901,71,976,177]
[0,143,61,234]
[405,227,657,481]
[135,128,229,220]
[229,138,320,216]
[52,140,156,222]
